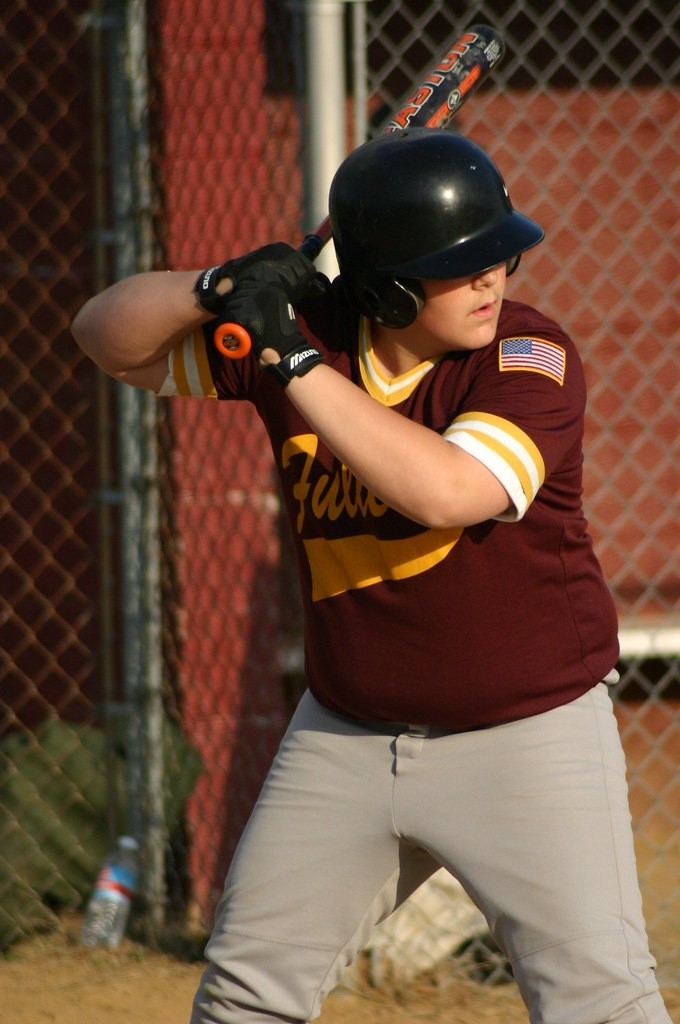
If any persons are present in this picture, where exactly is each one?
[72,127,674,1024]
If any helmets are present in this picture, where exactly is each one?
[330,127,545,330]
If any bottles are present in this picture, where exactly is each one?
[78,835,142,948]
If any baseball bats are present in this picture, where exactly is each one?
[214,23,507,358]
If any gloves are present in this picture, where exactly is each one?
[212,279,324,389]
[195,243,317,318]
